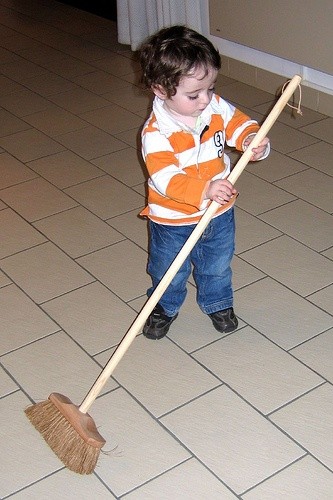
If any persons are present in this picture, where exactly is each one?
[139,24,271,340]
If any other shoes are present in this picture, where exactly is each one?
[207,306,238,334]
[142,303,179,340]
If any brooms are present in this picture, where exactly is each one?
[22,73,303,476]
